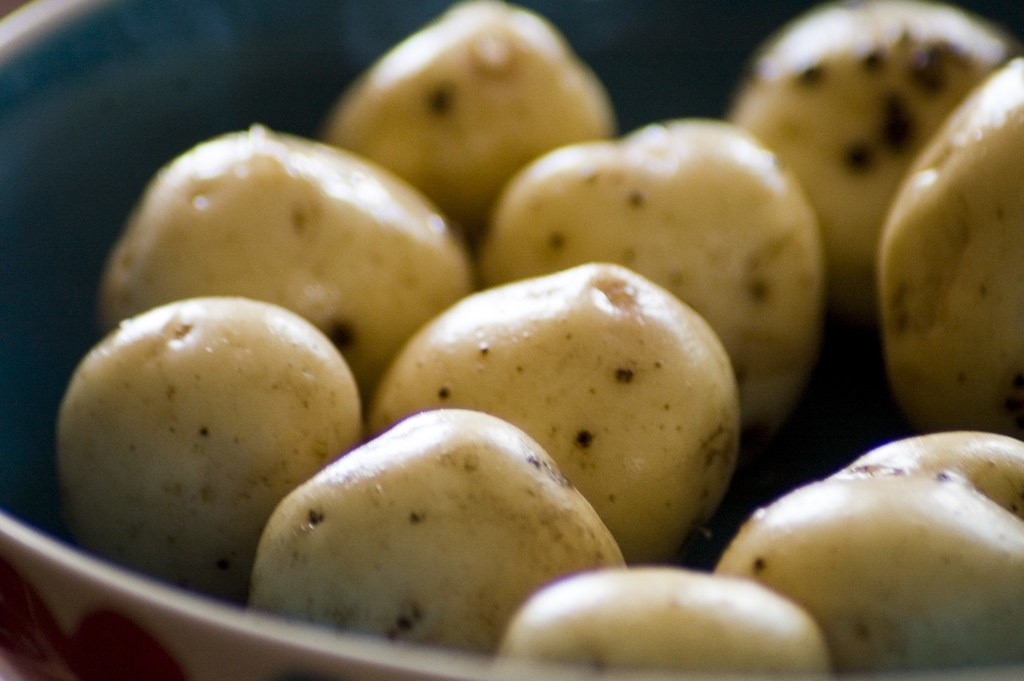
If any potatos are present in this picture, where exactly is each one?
[48,0,1024,681]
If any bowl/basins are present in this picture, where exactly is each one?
[0,0,1024,681]
